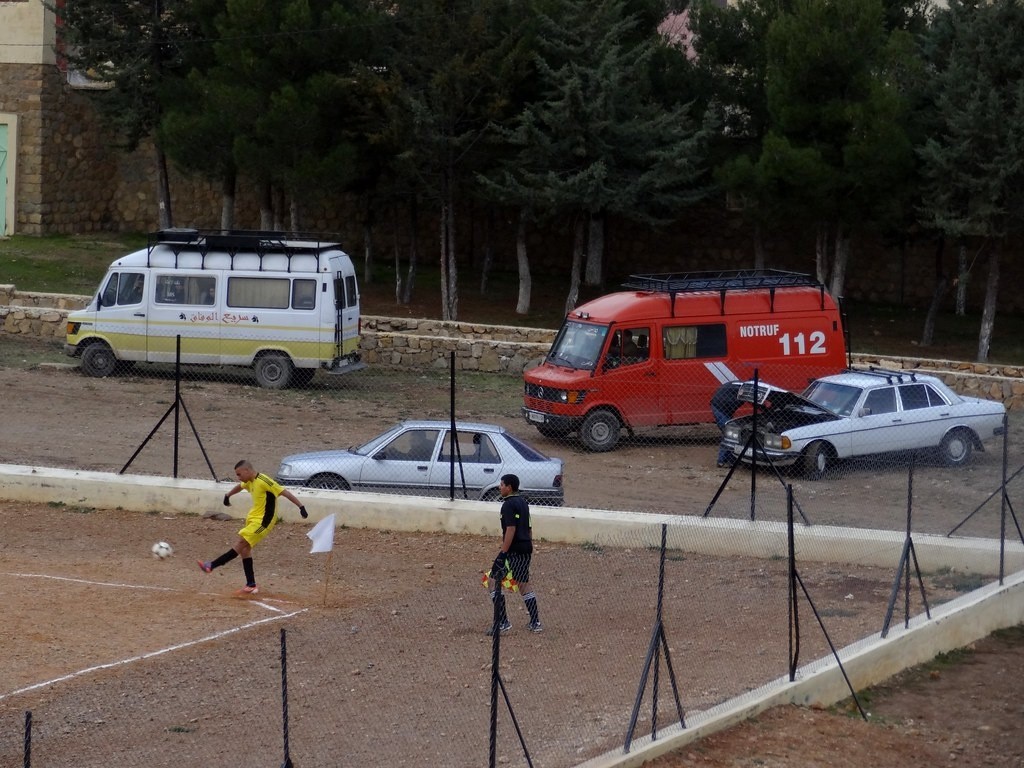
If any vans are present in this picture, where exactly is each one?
[65,227,368,389]
[521,265,853,452]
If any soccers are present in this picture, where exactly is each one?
[149,541,176,563]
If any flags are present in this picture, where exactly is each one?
[482,551,519,592]
[307,514,335,554]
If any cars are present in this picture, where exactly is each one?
[274,420,564,508]
[721,365,1007,478]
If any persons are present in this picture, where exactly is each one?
[196,460,308,595]
[388,429,434,461]
[613,330,639,365]
[484,473,543,635]
[203,284,215,305]
[710,377,767,467]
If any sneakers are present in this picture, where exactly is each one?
[231,586,262,600]
[196,559,212,574]
[521,622,543,634]
[485,618,512,636]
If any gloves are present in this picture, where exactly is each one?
[492,551,509,581]
[299,506,308,519]
[223,494,231,506]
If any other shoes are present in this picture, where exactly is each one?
[717,462,734,468]
